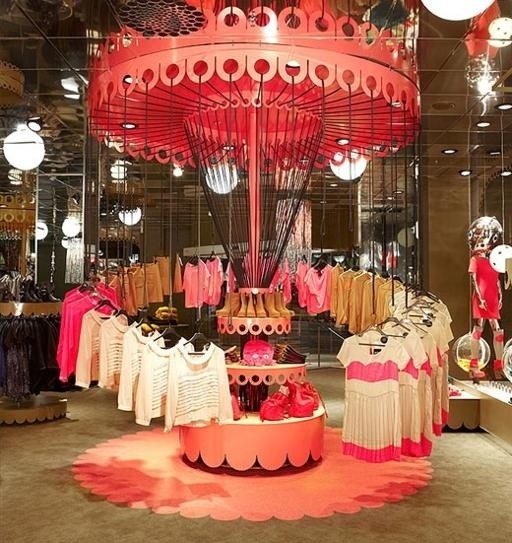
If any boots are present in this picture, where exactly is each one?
[0,275,61,303]
[216,291,295,319]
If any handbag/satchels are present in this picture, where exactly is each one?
[231,394,243,421]
[260,378,320,421]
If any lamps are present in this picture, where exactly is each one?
[72,193,82,204]
[26,116,45,132]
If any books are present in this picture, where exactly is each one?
[273,340,308,365]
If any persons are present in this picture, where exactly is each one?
[468,238,508,378]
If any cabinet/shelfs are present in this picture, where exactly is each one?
[181,289,326,471]
[1,300,68,425]
